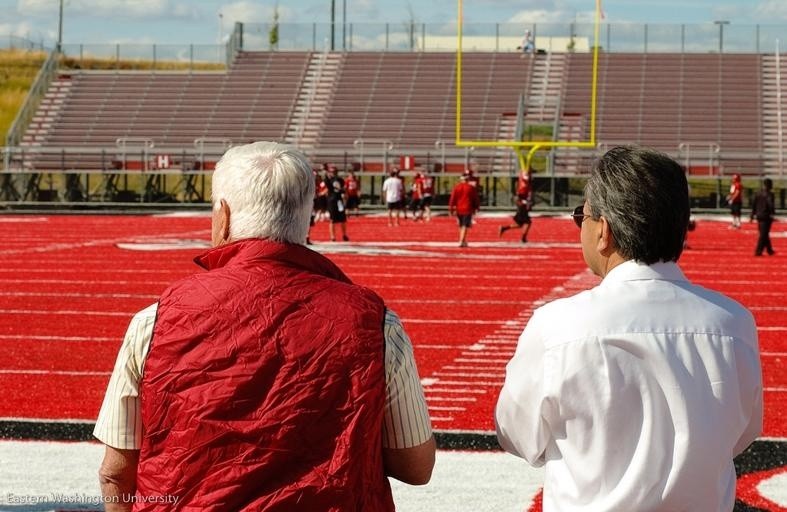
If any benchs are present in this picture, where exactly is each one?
[352,48,787,163]
[15,50,353,165]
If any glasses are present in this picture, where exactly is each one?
[571,206,600,228]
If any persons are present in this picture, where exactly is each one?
[519,29,535,56]
[304,166,777,255]
[90,139,435,511]
[492,145,762,511]
